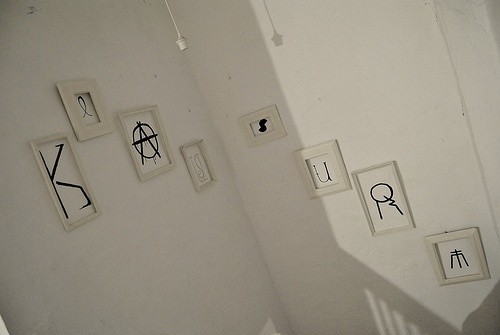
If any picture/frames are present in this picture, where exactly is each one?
[179,139,221,194]
[292,139,353,199]
[239,105,287,147]
[351,159,416,238]
[119,104,175,181]
[31,131,102,232]
[423,227,491,289]
[56,77,113,141]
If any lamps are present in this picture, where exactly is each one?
[164,0,187,51]
